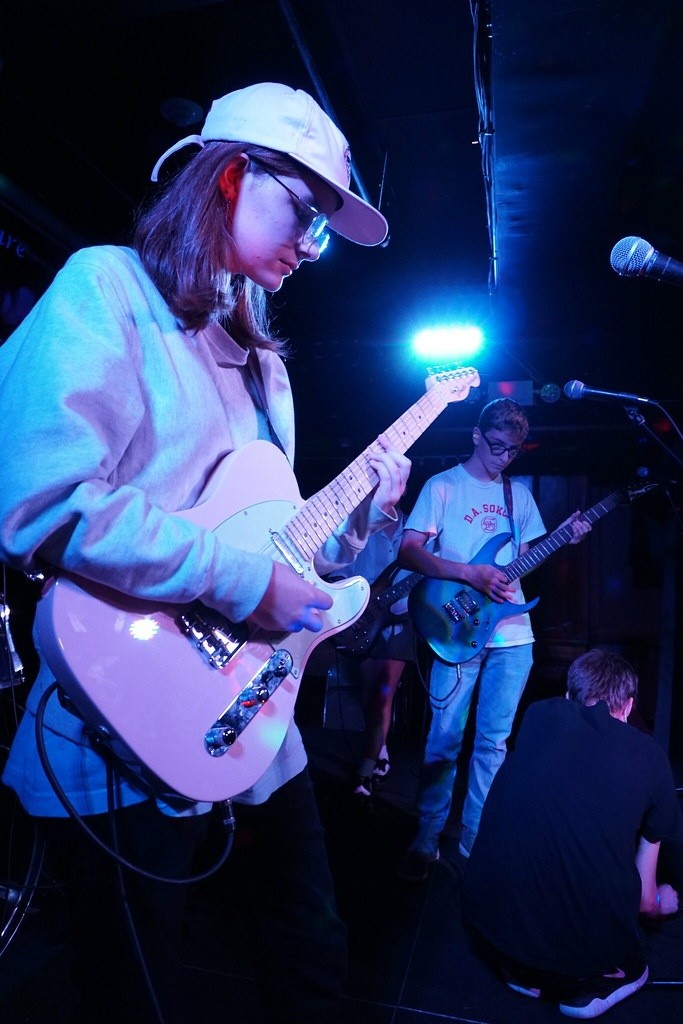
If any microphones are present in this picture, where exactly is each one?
[609,237,683,285]
[563,379,659,408]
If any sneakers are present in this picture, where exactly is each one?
[372,756,391,783]
[401,847,440,881]
[499,955,547,999]
[556,950,650,1019]
[352,777,373,798]
[458,840,474,861]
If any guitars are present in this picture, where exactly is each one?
[31,366,482,806]
[331,570,424,656]
[407,477,652,668]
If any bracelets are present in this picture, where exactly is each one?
[653,891,661,915]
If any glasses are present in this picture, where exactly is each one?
[477,430,521,458]
[244,153,332,254]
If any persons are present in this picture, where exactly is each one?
[395,397,592,885]
[462,647,679,1019]
[0,80,413,1023]
[313,482,435,796]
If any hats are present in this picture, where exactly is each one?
[151,83,390,248]
